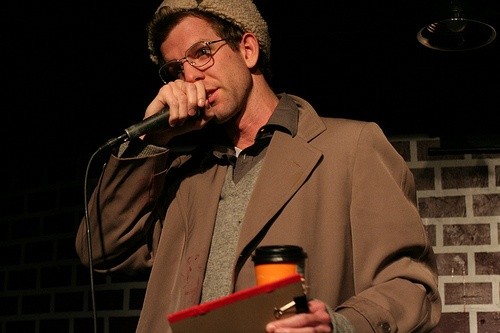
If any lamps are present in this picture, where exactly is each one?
[417,0,496,54]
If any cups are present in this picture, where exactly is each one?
[251,245,308,295]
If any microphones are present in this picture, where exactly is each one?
[99,106,205,151]
